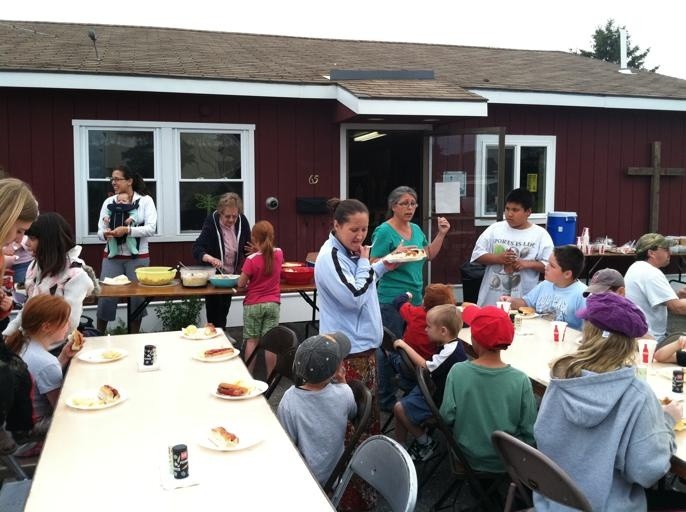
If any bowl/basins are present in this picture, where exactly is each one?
[134,265,177,286]
[279,262,313,284]
[180,266,240,288]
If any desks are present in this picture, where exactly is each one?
[81,273,320,331]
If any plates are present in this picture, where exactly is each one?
[63,325,271,452]
[385,256,424,262]
[100,280,131,285]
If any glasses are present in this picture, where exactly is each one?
[222,212,239,220]
[110,176,127,182]
[396,202,418,209]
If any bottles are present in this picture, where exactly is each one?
[504,248,516,272]
[577,227,590,254]
[172,444,189,480]
[672,371,683,393]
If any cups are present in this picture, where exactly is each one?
[582,244,606,255]
[497,302,658,367]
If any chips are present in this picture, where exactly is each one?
[181,325,199,338]
[100,349,121,359]
[72,397,97,407]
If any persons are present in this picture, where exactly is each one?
[1,211,98,383]
[394,304,467,461]
[471,188,554,306]
[95,165,158,337]
[313,187,418,509]
[583,233,686,369]
[392,283,454,361]
[500,246,589,330]
[0,176,40,314]
[371,188,450,435]
[239,220,286,391]
[280,332,361,487]
[6,198,40,287]
[102,192,140,259]
[193,193,252,332]
[531,292,682,510]
[440,304,537,512]
[3,295,88,442]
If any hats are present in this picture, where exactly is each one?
[635,233,679,256]
[292,331,352,382]
[574,291,648,339]
[462,305,515,351]
[584,267,625,298]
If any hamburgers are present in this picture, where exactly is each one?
[518,306,535,316]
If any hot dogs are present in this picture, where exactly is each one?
[99,384,120,404]
[210,426,237,447]
[203,347,235,358]
[203,322,217,337]
[218,382,251,396]
[67,329,85,351]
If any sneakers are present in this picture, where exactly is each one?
[411,440,440,464]
